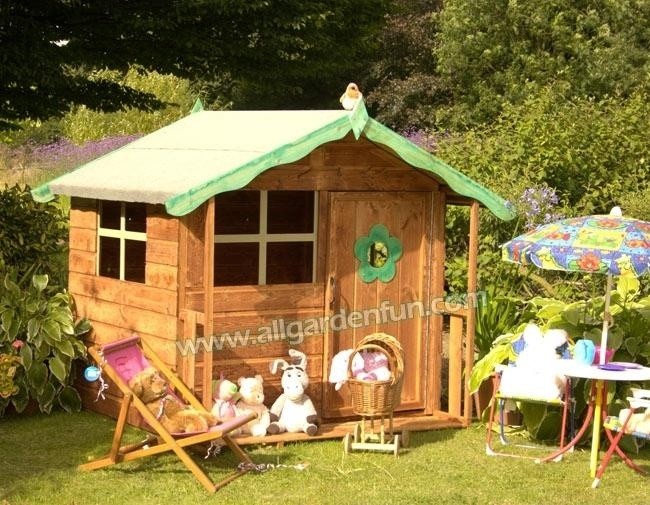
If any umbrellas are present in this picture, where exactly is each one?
[498,203,650,479]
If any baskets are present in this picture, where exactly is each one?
[347,333,405,416]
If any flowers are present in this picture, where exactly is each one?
[504,185,567,233]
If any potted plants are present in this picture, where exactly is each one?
[456,260,536,427]
[0,273,92,417]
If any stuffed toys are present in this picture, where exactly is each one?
[265,348,322,438]
[329,349,391,391]
[211,370,243,437]
[233,373,271,438]
[618,388,650,435]
[126,366,218,433]
[499,323,568,401]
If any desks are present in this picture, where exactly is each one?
[534,361,650,475]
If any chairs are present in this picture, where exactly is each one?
[487,319,580,462]
[589,389,650,492]
[78,335,260,495]
